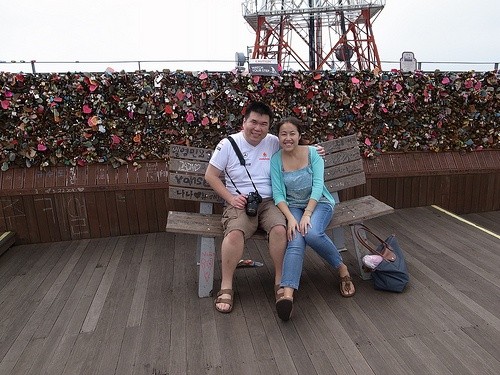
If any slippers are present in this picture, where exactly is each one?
[275,296,294,320]
[338,264,355,297]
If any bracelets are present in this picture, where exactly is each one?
[304,208,313,212]
[302,214,311,216]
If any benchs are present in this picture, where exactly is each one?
[166,134,395,297]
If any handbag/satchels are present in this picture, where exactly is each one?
[354,223,409,294]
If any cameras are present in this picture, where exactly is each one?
[243,192,262,217]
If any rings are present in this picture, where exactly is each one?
[299,224,302,226]
[309,224,312,229]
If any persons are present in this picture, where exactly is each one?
[270,117,357,321]
[204,101,326,314]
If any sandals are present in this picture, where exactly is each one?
[214,289,234,312]
[275,284,284,300]
